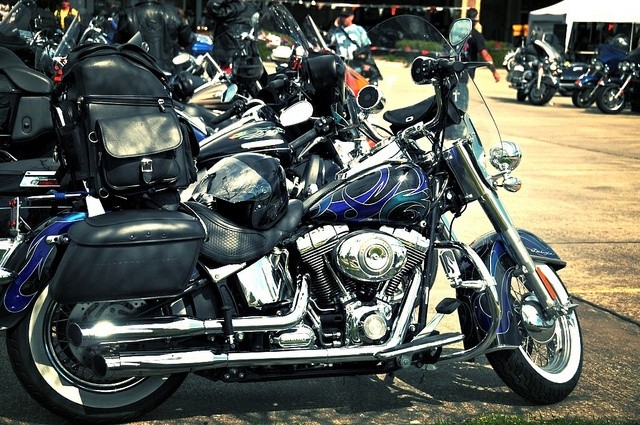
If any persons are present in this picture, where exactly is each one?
[206,0,264,98]
[322,10,371,80]
[112,0,182,69]
[52,0,80,30]
[462,8,500,84]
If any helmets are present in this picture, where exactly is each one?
[611,33,629,52]
[190,153,288,231]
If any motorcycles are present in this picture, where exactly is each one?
[503,25,639,116]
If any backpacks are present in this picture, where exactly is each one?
[49,44,199,200]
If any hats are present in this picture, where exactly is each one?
[340,8,353,16]
